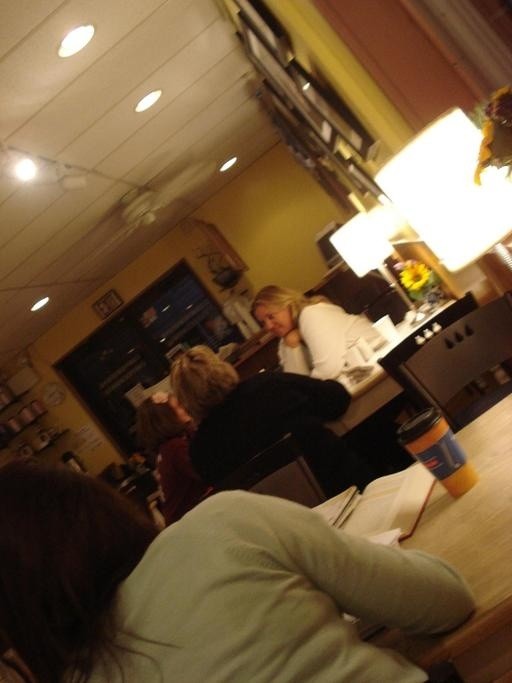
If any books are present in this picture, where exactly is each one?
[310,458,438,543]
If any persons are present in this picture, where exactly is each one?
[130,391,209,520]
[251,284,385,382]
[1,459,477,683]
[170,344,352,487]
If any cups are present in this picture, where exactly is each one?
[395,404,479,499]
[370,312,402,349]
